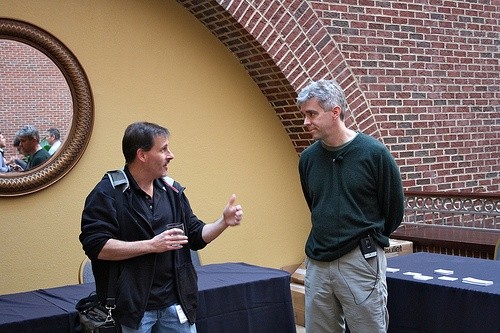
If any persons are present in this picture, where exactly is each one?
[79,122,243,333]
[46,127,61,156]
[18,124,51,171]
[13,137,30,163]
[0,131,21,172]
[296,80,404,333]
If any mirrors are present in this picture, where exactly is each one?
[0,17,95,198]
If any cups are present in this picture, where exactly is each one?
[165,222,185,247]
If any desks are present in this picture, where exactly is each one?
[387,252,500,333]
[0,262,298,333]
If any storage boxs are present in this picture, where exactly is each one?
[384,238,414,258]
[280,261,306,327]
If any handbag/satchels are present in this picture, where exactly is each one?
[76,294,120,333]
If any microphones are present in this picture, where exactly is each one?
[332,156,343,162]
